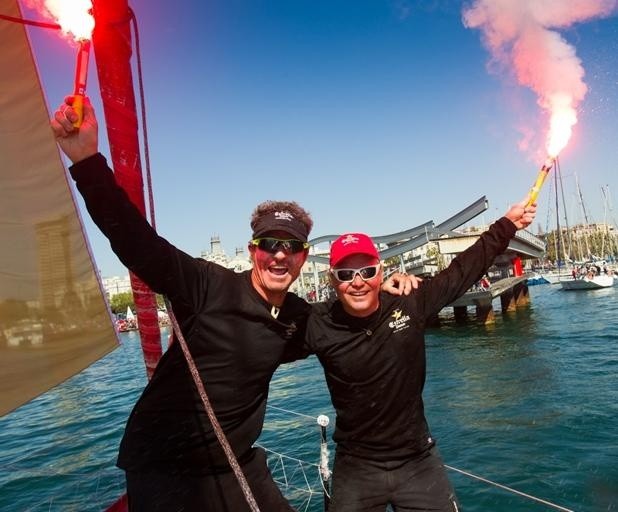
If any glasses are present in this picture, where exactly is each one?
[329,264,380,283]
[250,237,309,254]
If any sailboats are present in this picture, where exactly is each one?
[510,151,618,291]
[113,305,173,331]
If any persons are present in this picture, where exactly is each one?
[571,261,614,280]
[52,94,424,512]
[307,188,537,512]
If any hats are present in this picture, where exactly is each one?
[330,233,378,266]
[252,210,308,243]
